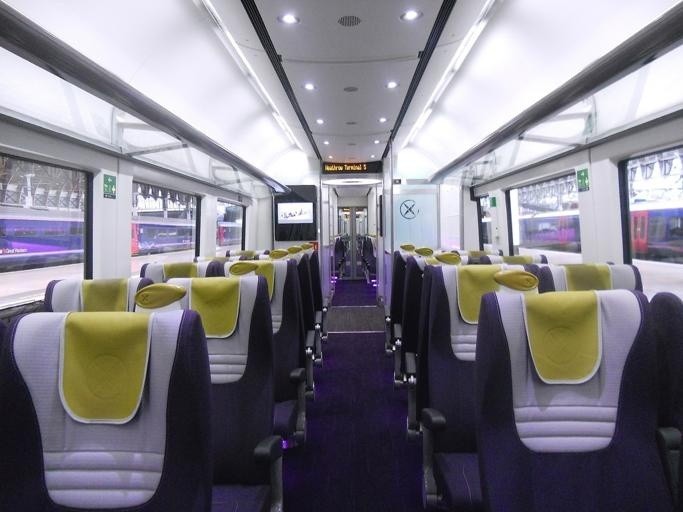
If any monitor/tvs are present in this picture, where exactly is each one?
[278,202,315,225]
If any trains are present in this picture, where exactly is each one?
[481,203,683,263]
[0,202,241,273]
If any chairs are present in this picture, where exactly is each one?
[140,246,330,449]
[332,231,350,280]
[420,262,683,507]
[389,245,615,439]
[358,233,378,285]
[4,274,287,509]
